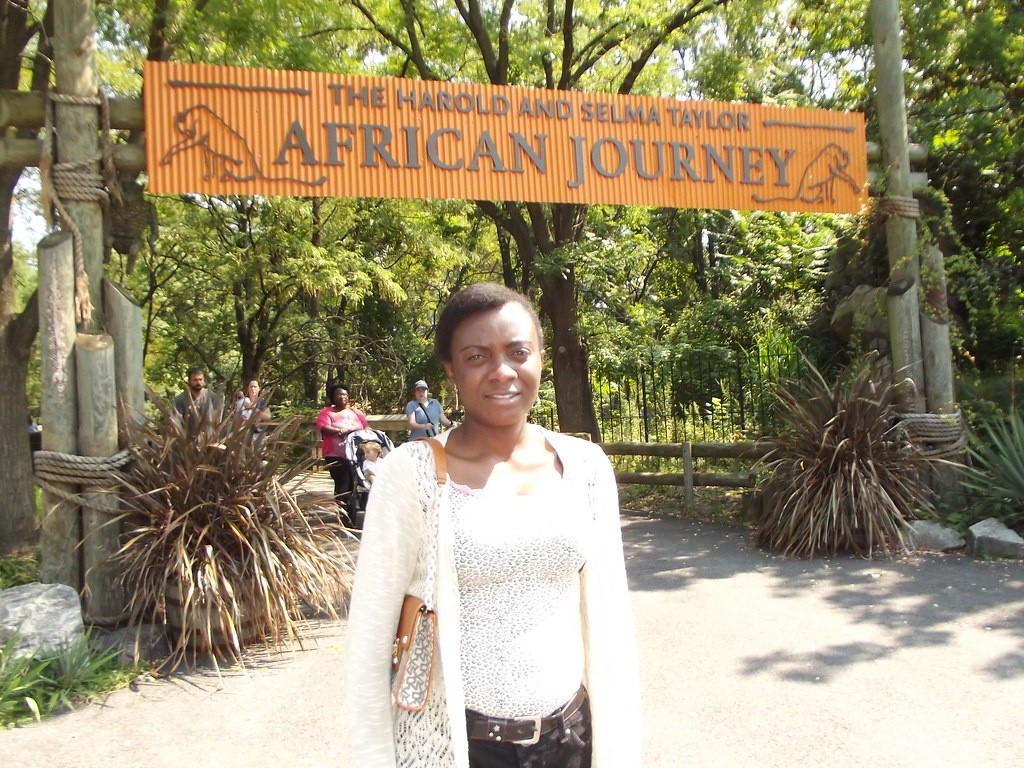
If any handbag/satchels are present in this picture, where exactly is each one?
[391,437,449,711]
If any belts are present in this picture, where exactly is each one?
[469,687,585,746]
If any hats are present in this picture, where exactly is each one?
[415,380,428,390]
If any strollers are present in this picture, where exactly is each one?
[338,428,397,528]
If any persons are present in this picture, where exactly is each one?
[406,379,458,441]
[317,385,369,525]
[173,367,271,461]
[362,441,383,484]
[344,283,641,768]
